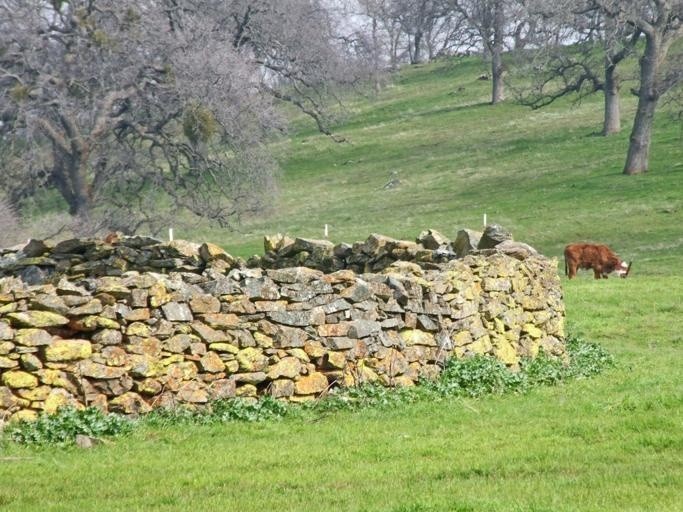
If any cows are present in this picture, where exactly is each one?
[563,243,628,279]
